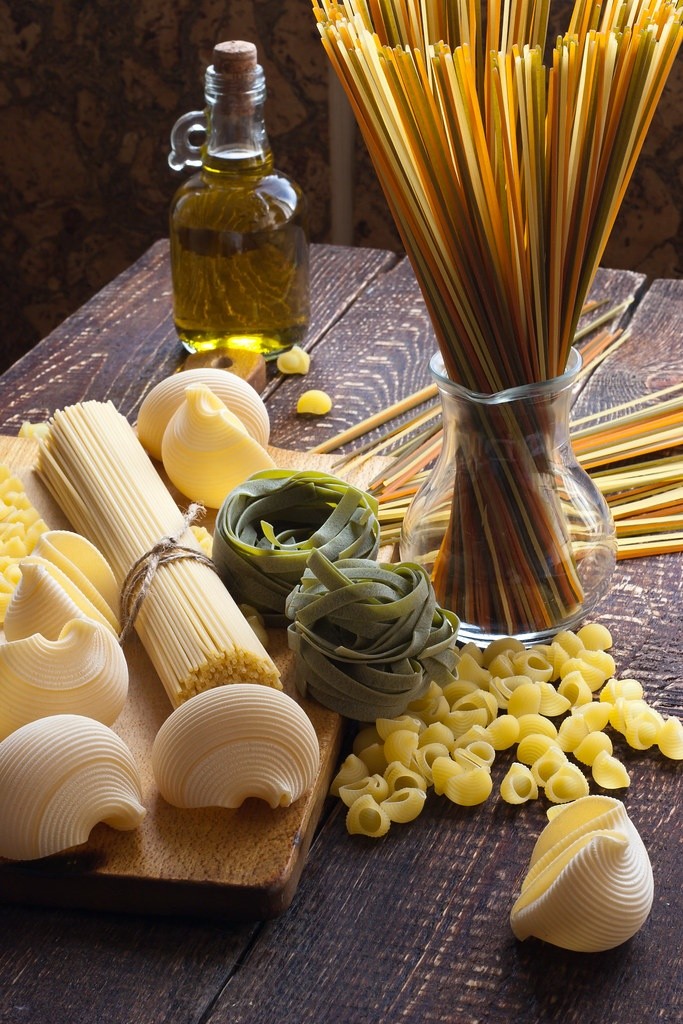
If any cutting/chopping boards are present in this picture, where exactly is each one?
[0,437,398,912]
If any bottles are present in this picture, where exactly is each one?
[168,41,310,361]
[400,347,618,651]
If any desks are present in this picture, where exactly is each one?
[0,237,682,1024]
